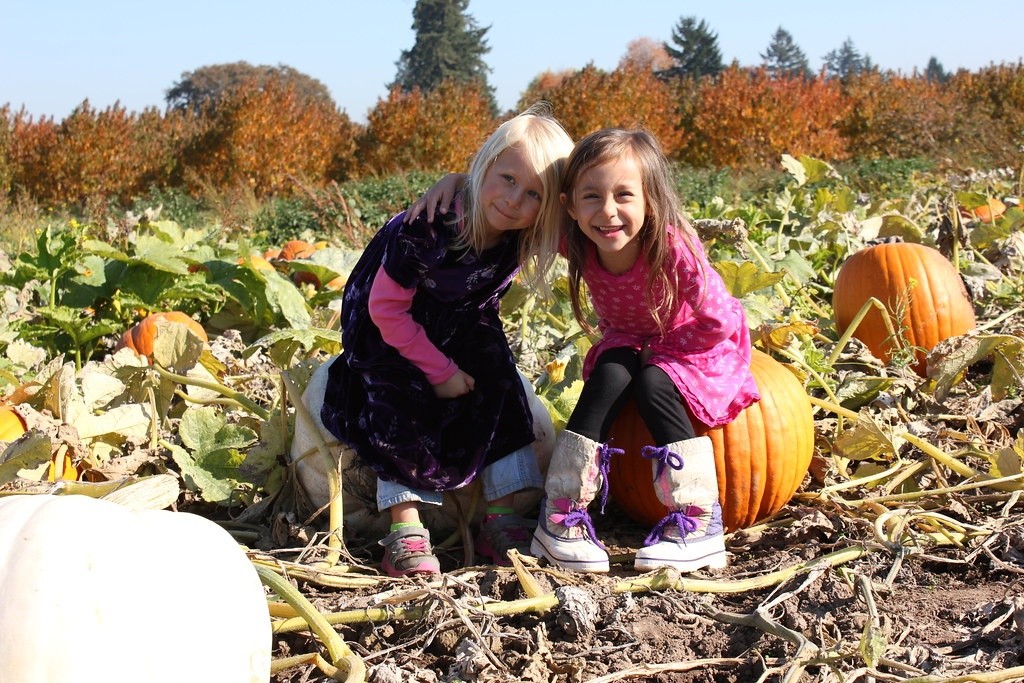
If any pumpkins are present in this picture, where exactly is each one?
[961,197,1006,224]
[116,312,208,365]
[48,449,82,482]
[0,406,27,442]
[606,345,814,530]
[288,347,554,531]
[833,236,975,377]
[0,477,272,683]
[188,241,347,290]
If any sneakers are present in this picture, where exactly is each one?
[473,512,548,568]
[377,525,441,578]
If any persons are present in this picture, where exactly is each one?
[319,115,575,575]
[403,129,763,573]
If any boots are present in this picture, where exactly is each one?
[529,427,626,574]
[633,435,727,574]
